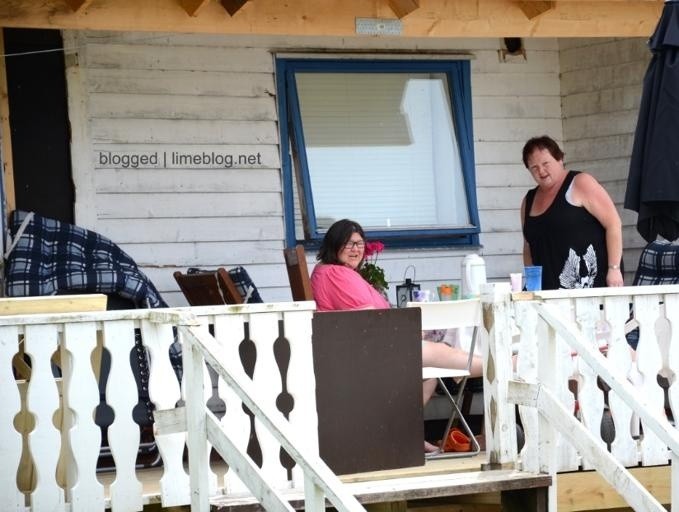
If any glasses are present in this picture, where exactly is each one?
[342,241,366,249]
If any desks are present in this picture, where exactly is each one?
[403,292,514,461]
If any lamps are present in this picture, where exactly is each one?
[397,265,422,308]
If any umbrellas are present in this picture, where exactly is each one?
[622,2,678,245]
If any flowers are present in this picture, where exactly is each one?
[355,242,389,288]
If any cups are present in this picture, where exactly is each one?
[510,272,522,292]
[524,266,542,291]
[411,290,434,302]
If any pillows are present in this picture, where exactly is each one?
[187,266,264,303]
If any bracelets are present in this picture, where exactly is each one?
[607,261,624,271]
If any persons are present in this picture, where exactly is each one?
[519,135,627,287]
[309,220,522,452]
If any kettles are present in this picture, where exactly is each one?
[459,253,487,299]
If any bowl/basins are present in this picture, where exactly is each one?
[436,284,459,302]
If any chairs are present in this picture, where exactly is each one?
[404,297,491,457]
[280,242,314,302]
[623,235,679,348]
[173,269,245,307]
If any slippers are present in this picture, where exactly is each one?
[435,430,472,452]
